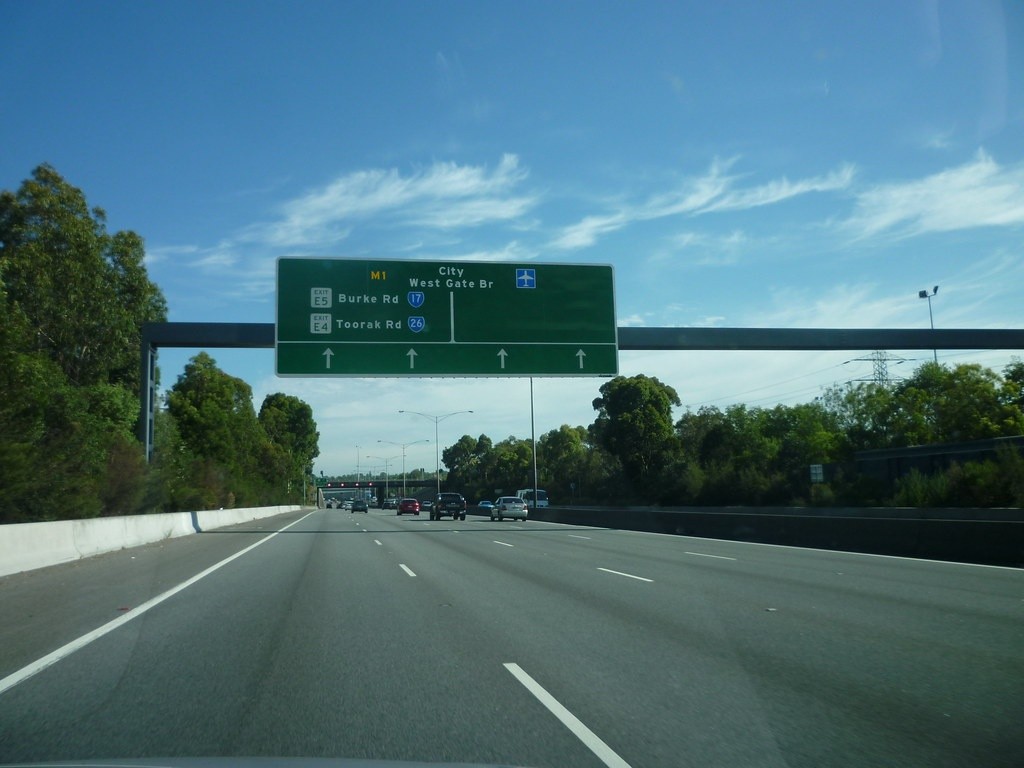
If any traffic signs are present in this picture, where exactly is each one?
[272,254,620,380]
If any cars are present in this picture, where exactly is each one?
[421,501,432,510]
[382,501,393,510]
[478,500,493,508]
[489,497,529,522]
[324,496,369,513]
[396,498,420,515]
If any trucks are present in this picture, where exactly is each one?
[515,488,550,509]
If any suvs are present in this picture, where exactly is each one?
[429,493,467,521]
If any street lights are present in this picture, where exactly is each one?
[917,285,941,365]
[397,409,475,494]
[356,464,392,496]
[355,444,361,481]
[377,439,430,497]
[366,455,408,499]
